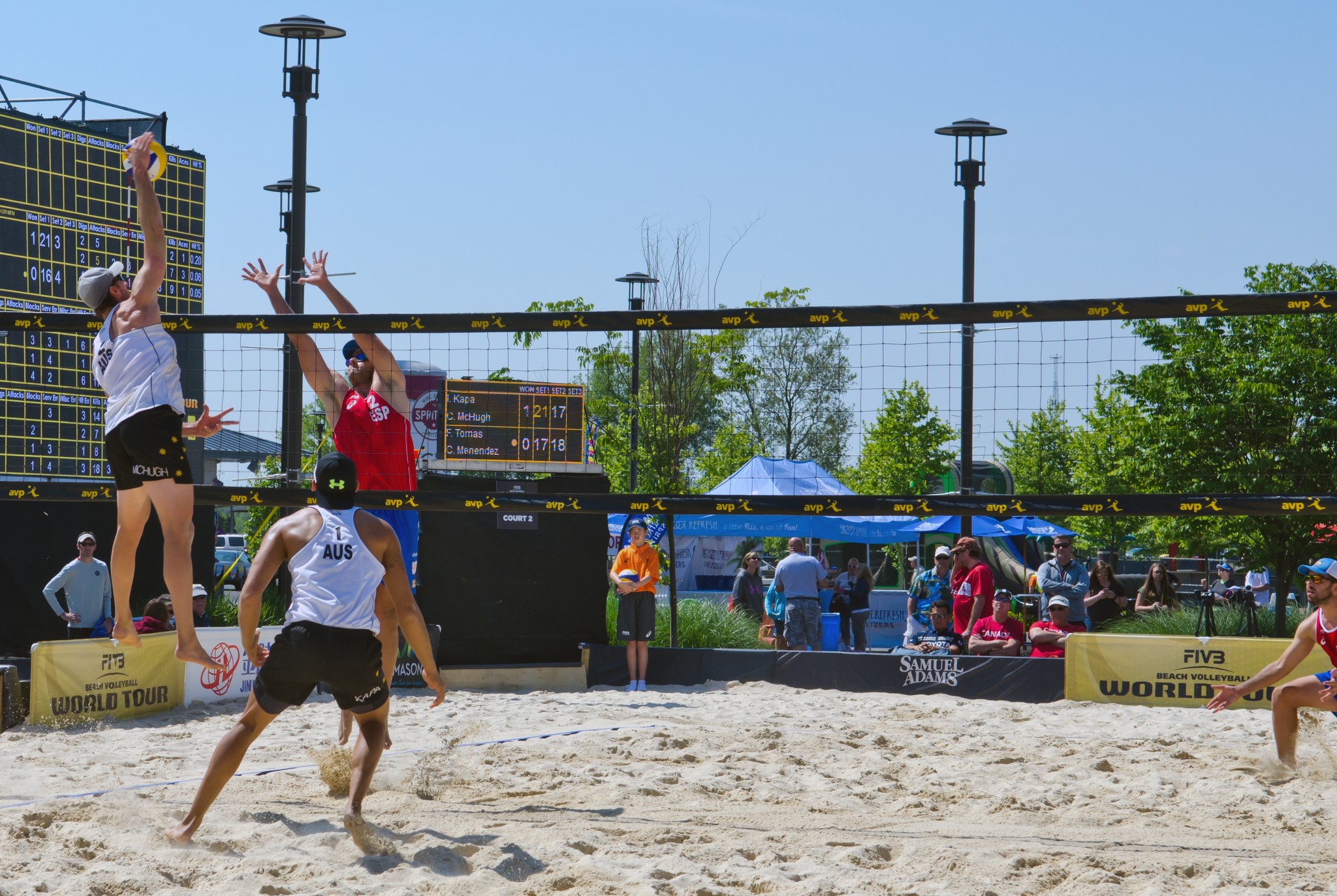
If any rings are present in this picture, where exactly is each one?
[1100,594,1102,596]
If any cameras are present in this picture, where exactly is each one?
[1200,590,1215,600]
[1242,585,1254,600]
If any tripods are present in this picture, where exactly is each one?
[1236,601,1261,637]
[1194,599,1218,637]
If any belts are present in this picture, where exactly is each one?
[786,597,817,601]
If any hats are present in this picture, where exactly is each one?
[1216,564,1233,575]
[1047,595,1069,608]
[1298,558,1337,579]
[78,261,124,310]
[342,339,363,360]
[949,537,977,552]
[193,584,208,597]
[935,546,951,557]
[316,453,356,510]
[628,518,647,531]
[77,534,96,544]
[994,589,1012,601]
[158,594,172,606]
[908,556,920,564]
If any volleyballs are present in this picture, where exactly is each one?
[122,135,167,182]
[618,569,639,591]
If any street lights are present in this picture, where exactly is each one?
[933,117,1008,543]
[257,13,347,605]
[614,271,660,494]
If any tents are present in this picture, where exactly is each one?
[702,453,921,574]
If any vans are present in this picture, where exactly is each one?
[215,533,250,554]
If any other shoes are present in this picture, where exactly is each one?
[626,680,647,692]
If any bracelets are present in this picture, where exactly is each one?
[1111,595,1117,600]
[1095,594,1099,601]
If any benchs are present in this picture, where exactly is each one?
[1115,574,1149,613]
[956,640,1032,657]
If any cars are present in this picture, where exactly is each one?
[214,550,252,591]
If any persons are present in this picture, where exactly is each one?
[43,531,113,640]
[1200,556,1270,608]
[239,249,420,750]
[609,518,660,693]
[1027,533,1128,658]
[968,588,1024,657]
[1206,558,1337,770]
[165,453,447,844]
[902,536,995,655]
[732,537,829,651]
[1134,555,1181,613]
[833,558,875,653]
[72,131,229,672]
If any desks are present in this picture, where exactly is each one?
[1011,593,1042,621]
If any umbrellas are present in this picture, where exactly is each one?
[892,515,1027,544]
[1001,516,1082,630]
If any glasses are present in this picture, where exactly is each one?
[80,542,95,547]
[936,556,949,560]
[750,557,761,561]
[113,274,124,285]
[1054,543,1069,549]
[1050,606,1064,611]
[995,596,1009,602]
[848,565,858,568]
[930,613,948,619]
[1303,574,1332,584]
[955,550,961,556]
[193,595,207,600]
[346,353,368,366]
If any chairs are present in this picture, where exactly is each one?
[758,601,775,639]
